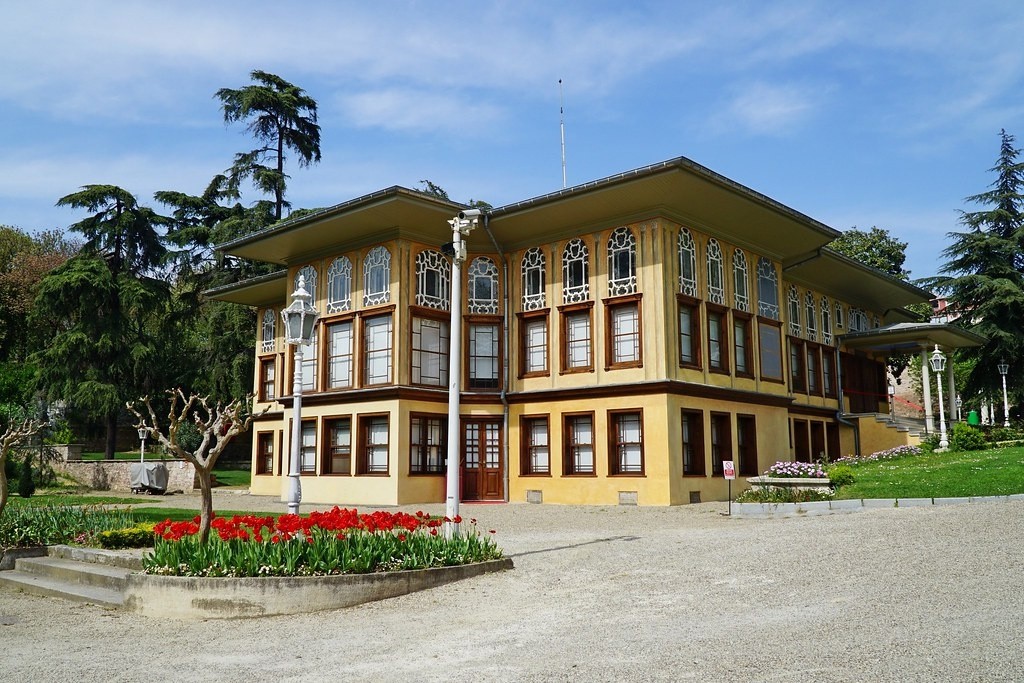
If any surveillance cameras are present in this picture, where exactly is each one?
[456,209,481,224]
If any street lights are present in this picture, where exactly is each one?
[955,395,963,422]
[138,427,150,466]
[928,343,950,450]
[996,355,1012,428]
[280,274,322,516]
